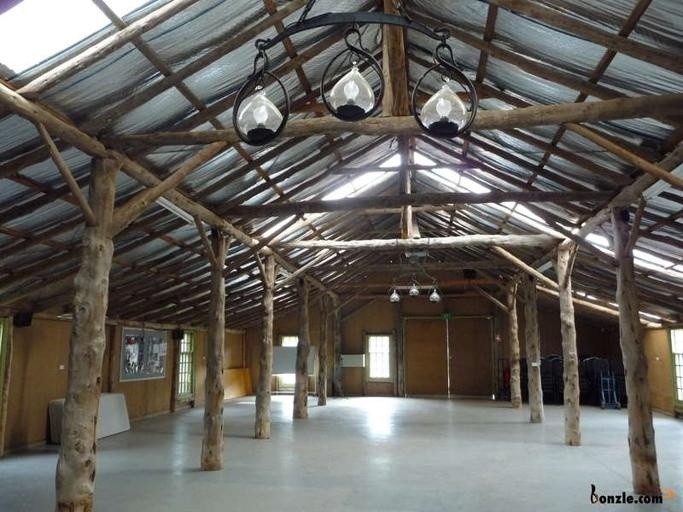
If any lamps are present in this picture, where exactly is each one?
[231,0,478,146]
[382,250,445,305]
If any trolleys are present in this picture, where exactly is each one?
[593,356,623,410]
